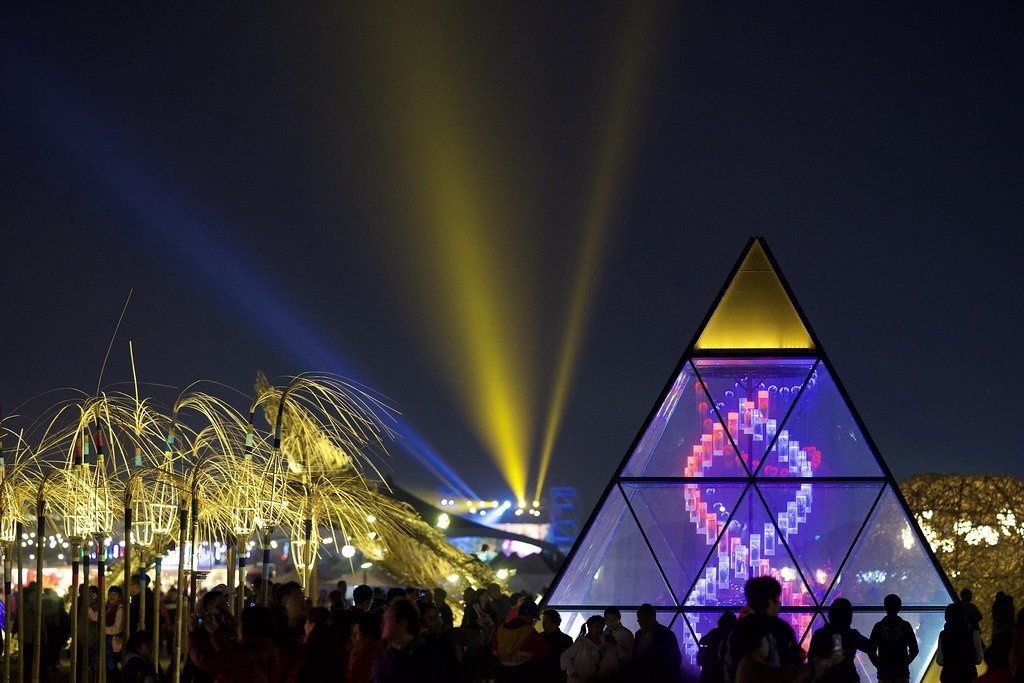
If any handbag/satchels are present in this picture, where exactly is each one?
[467,604,495,646]
[599,644,619,677]
[697,642,708,666]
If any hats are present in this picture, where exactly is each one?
[212,584,230,595]
[521,600,540,621]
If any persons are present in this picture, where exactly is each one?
[0,576,1024,683]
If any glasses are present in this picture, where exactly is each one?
[424,611,442,625]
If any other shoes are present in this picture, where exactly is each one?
[51,668,60,673]
